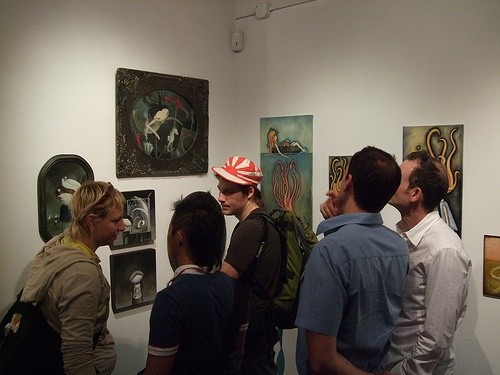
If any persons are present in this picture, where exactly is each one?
[132,191,249,375]
[19,181,126,375]
[211,156,287,375]
[374,151,472,375]
[294,146,410,375]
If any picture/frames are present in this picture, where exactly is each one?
[37,155,97,243]
[483,235,500,299]
[108,190,156,250]
[109,247,157,315]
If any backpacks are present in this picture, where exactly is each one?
[0,285,101,375]
[232,207,319,328]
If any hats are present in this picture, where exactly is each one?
[212,156,264,187]
